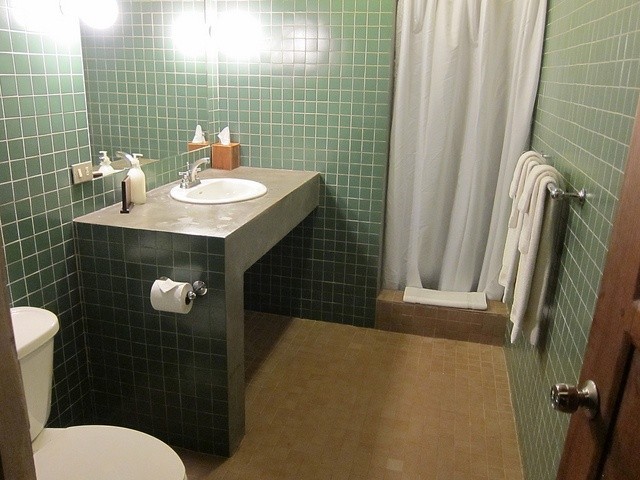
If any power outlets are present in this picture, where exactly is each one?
[73,161,93,186]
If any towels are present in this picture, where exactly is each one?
[403,286,487,311]
[503,163,563,348]
[497,151,548,305]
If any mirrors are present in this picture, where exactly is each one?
[76,0,211,181]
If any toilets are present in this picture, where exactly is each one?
[11,306,187,480]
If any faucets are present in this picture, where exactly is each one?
[116,151,132,168]
[177,157,211,188]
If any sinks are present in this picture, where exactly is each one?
[169,178,267,204]
